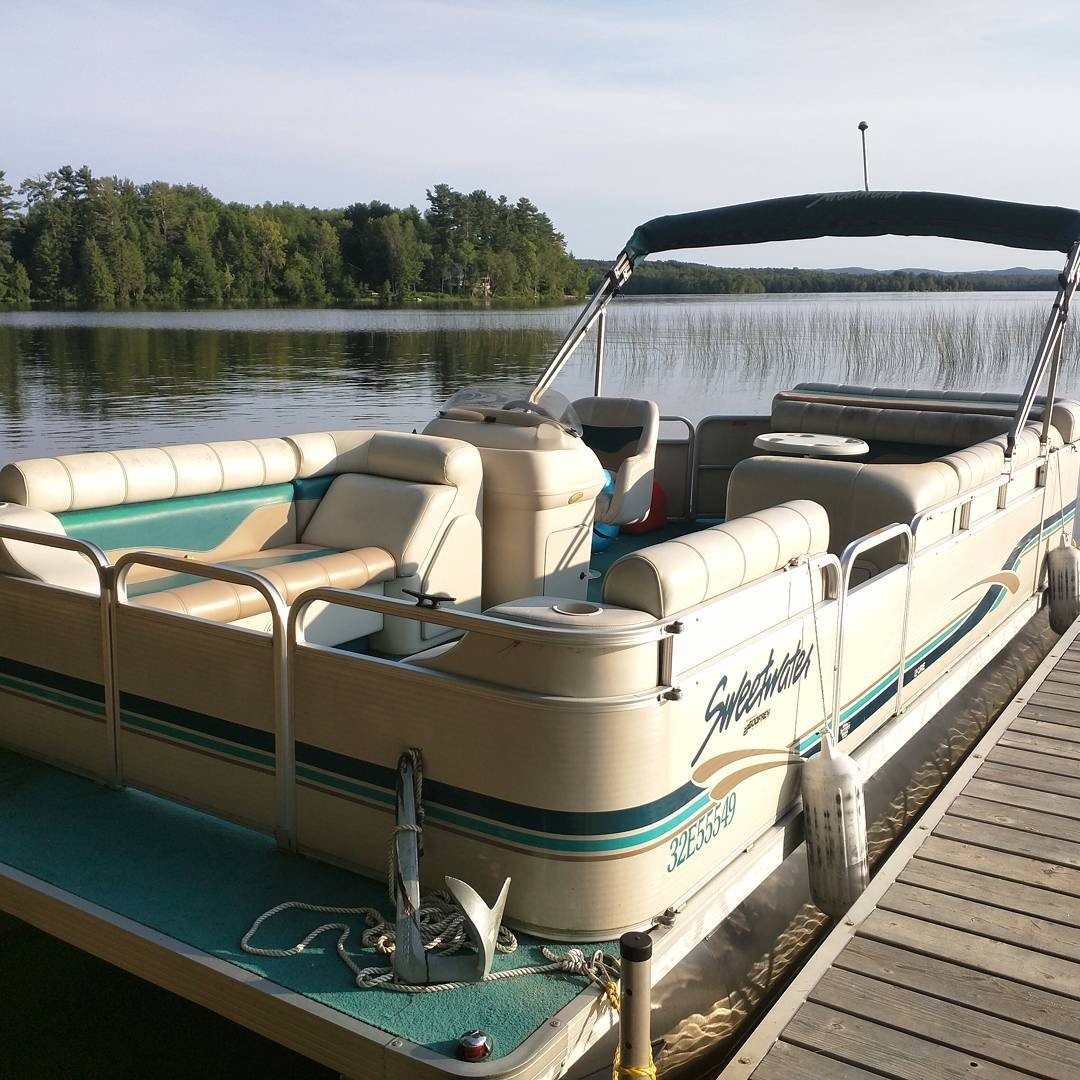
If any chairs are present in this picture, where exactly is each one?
[555,393,661,531]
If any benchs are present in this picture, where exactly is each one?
[592,489,833,673]
[721,386,1079,562]
[2,428,487,660]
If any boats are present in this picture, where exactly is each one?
[1,120,1080,1079]
